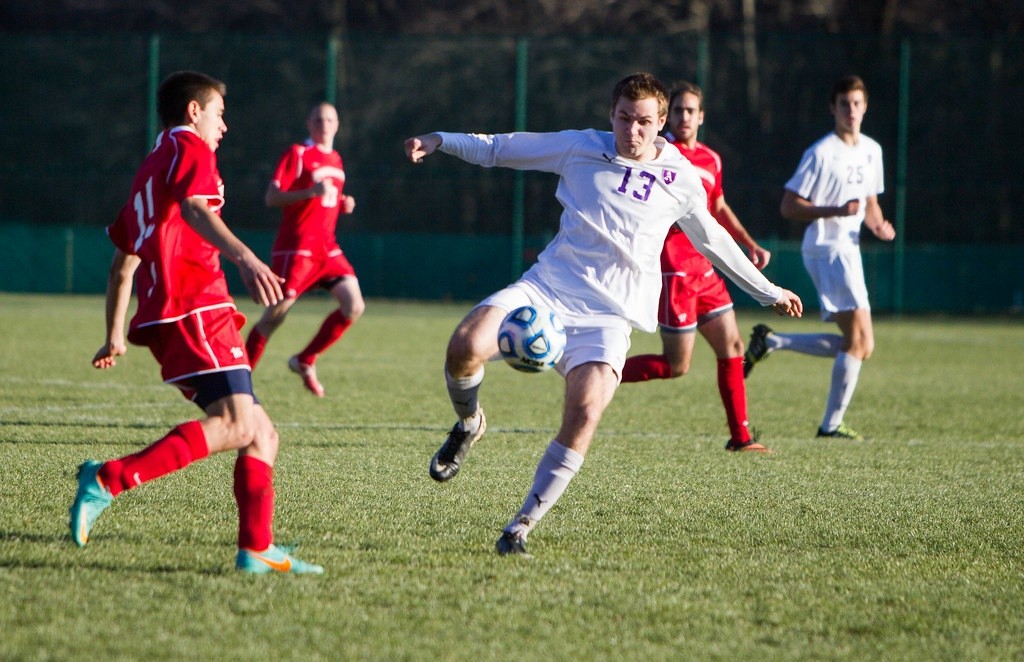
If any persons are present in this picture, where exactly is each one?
[405,73,803,556]
[744,75,896,442]
[246,104,364,398]
[69,71,322,575]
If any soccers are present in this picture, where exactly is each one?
[497,305,568,373]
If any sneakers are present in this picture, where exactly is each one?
[67,460,113,550]
[743,324,772,378]
[236,542,323,577]
[817,421,864,441]
[429,408,487,481]
[726,427,775,454]
[495,530,535,560]
[288,356,324,399]
